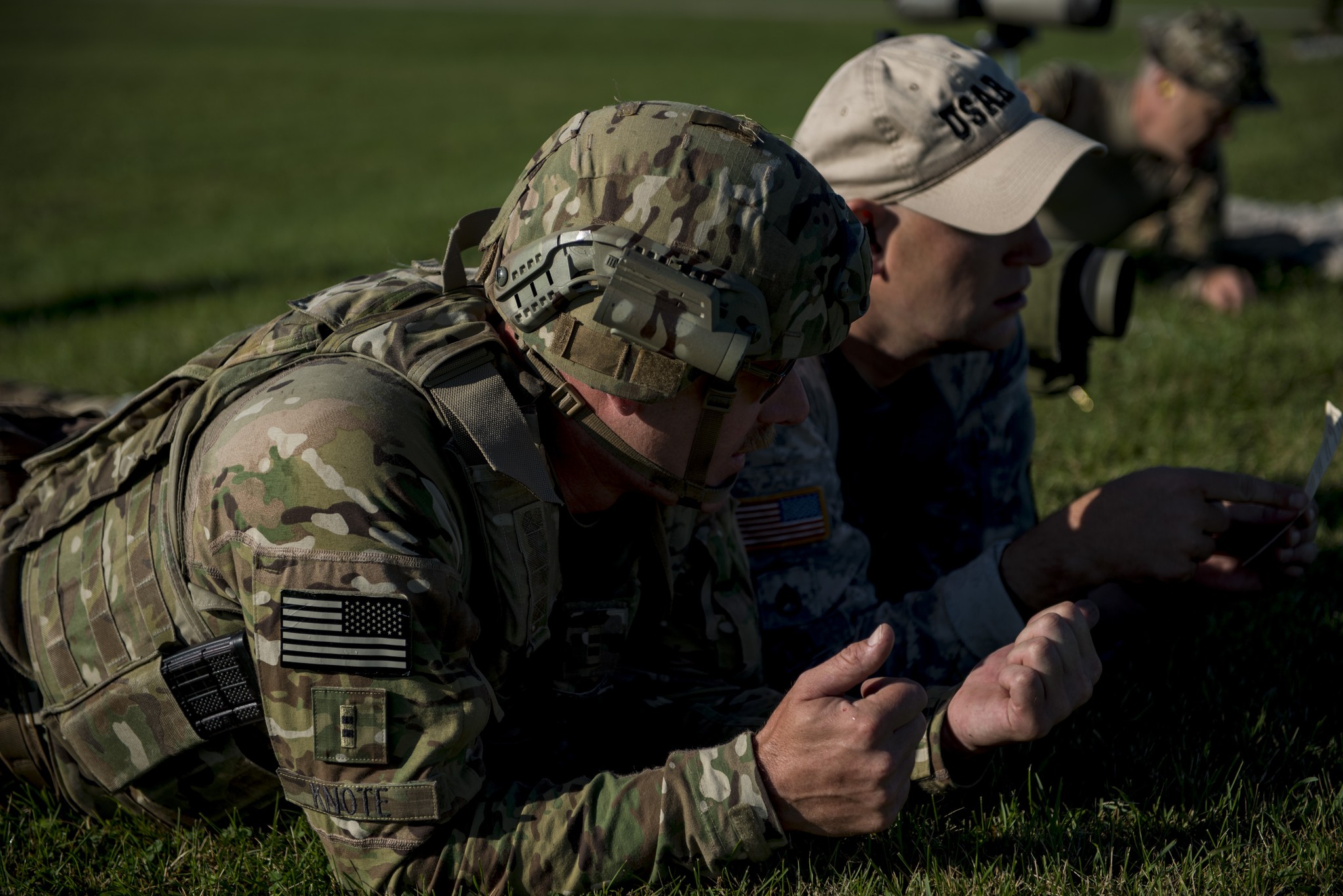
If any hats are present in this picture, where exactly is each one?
[790,34,1110,237]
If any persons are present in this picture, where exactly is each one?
[735,35,1320,684]
[1014,16,1289,311]
[1,101,1107,896]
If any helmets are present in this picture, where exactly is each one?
[476,101,876,407]
[1145,6,1278,108]
